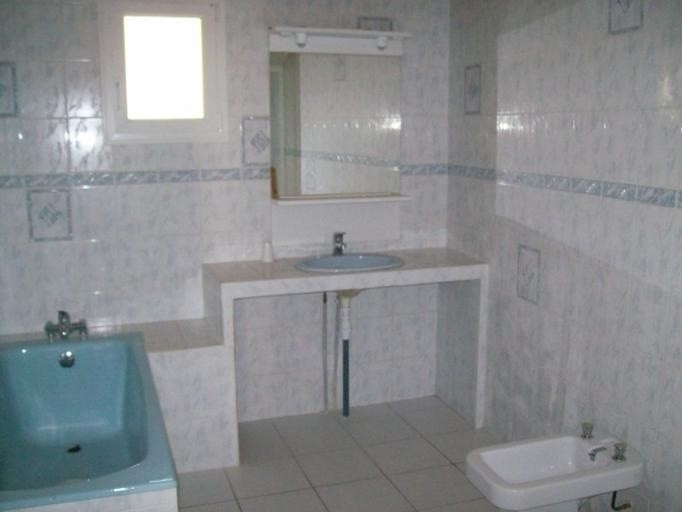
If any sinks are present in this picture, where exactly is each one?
[464,429,644,511]
[295,254,403,274]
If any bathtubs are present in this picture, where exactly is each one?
[0,331,179,512]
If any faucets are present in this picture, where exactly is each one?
[333,232,347,256]
[586,437,623,462]
[44,311,88,344]
